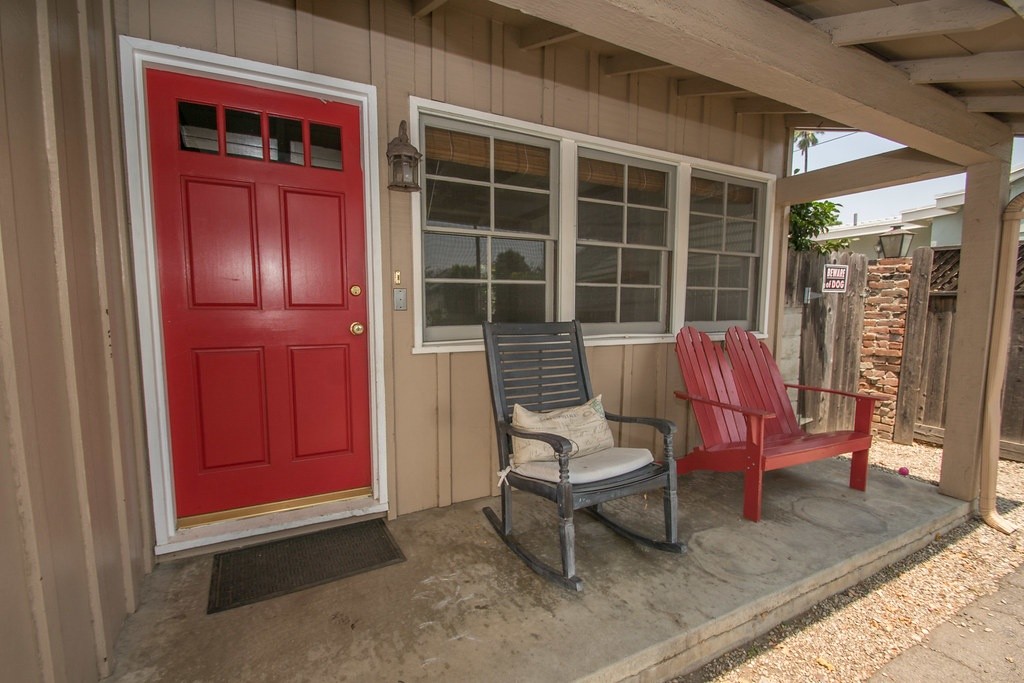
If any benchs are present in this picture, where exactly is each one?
[655,325,891,523]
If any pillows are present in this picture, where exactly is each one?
[508,392,615,467]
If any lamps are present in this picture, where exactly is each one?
[386,119,423,194]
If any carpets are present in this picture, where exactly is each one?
[205,517,407,615]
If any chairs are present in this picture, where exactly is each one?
[481,319,687,592]
